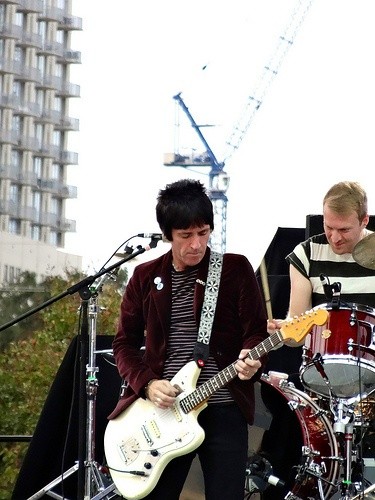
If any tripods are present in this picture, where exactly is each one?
[0,246,160,500]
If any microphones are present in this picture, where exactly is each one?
[244,460,265,476]
[138,233,168,243]
[320,273,333,300]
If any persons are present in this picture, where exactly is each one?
[112,178,266,500]
[266,180,374,490]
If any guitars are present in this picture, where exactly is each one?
[102,307,330,500]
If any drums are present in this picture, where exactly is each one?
[298,300,375,402]
[245,369,343,500]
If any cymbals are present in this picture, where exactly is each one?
[351,232,375,271]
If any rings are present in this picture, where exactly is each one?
[246,371,249,376]
